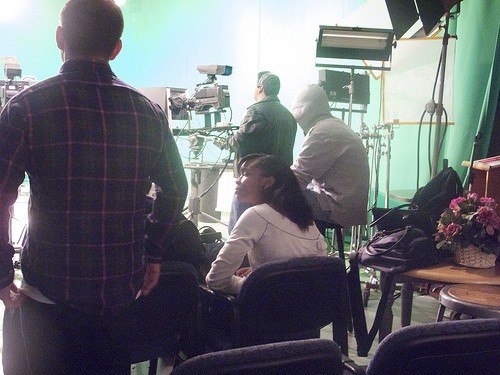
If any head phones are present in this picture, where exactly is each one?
[258,73,278,88]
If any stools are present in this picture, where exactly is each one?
[314,220,352,261]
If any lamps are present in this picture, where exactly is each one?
[318,69,372,104]
[384,0,463,40]
[314,24,394,61]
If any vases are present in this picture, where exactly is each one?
[452,244,498,269]
[432,191,500,258]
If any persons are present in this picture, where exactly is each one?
[221,71,297,276]
[205,152,327,293]
[289,84,368,227]
[0,0,199,375]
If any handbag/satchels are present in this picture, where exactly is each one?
[369,203,436,239]
[356,226,439,273]
[410,158,464,223]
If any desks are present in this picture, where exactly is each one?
[185,162,233,224]
[379,260,500,344]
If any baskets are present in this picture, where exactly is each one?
[454,244,496,268]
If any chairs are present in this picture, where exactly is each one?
[130,262,201,375]
[173,256,348,369]
[358,318,500,375]
[171,338,365,375]
